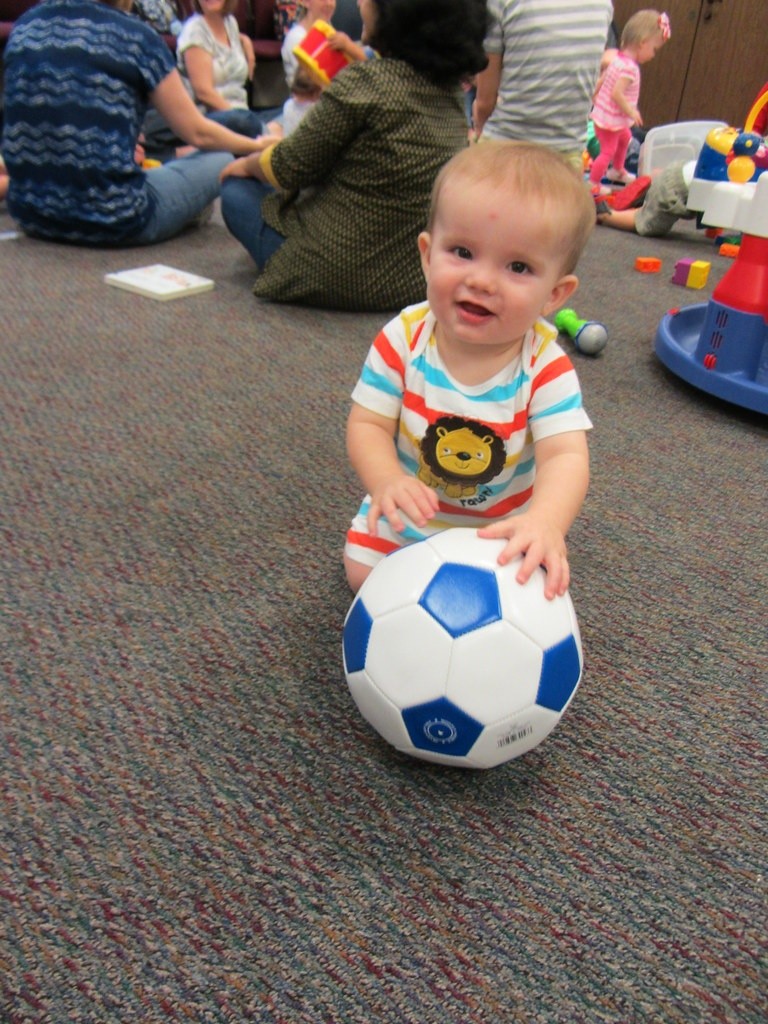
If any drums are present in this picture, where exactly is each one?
[291,18,354,87]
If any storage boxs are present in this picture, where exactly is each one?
[638,119,734,188]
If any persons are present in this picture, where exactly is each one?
[218,0,493,315]
[342,139,596,602]
[1,0,638,193]
[471,0,615,165]
[1,0,278,249]
[587,9,670,194]
[598,159,718,238]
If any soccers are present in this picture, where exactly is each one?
[338,526,585,772]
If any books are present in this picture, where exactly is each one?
[103,264,216,301]
